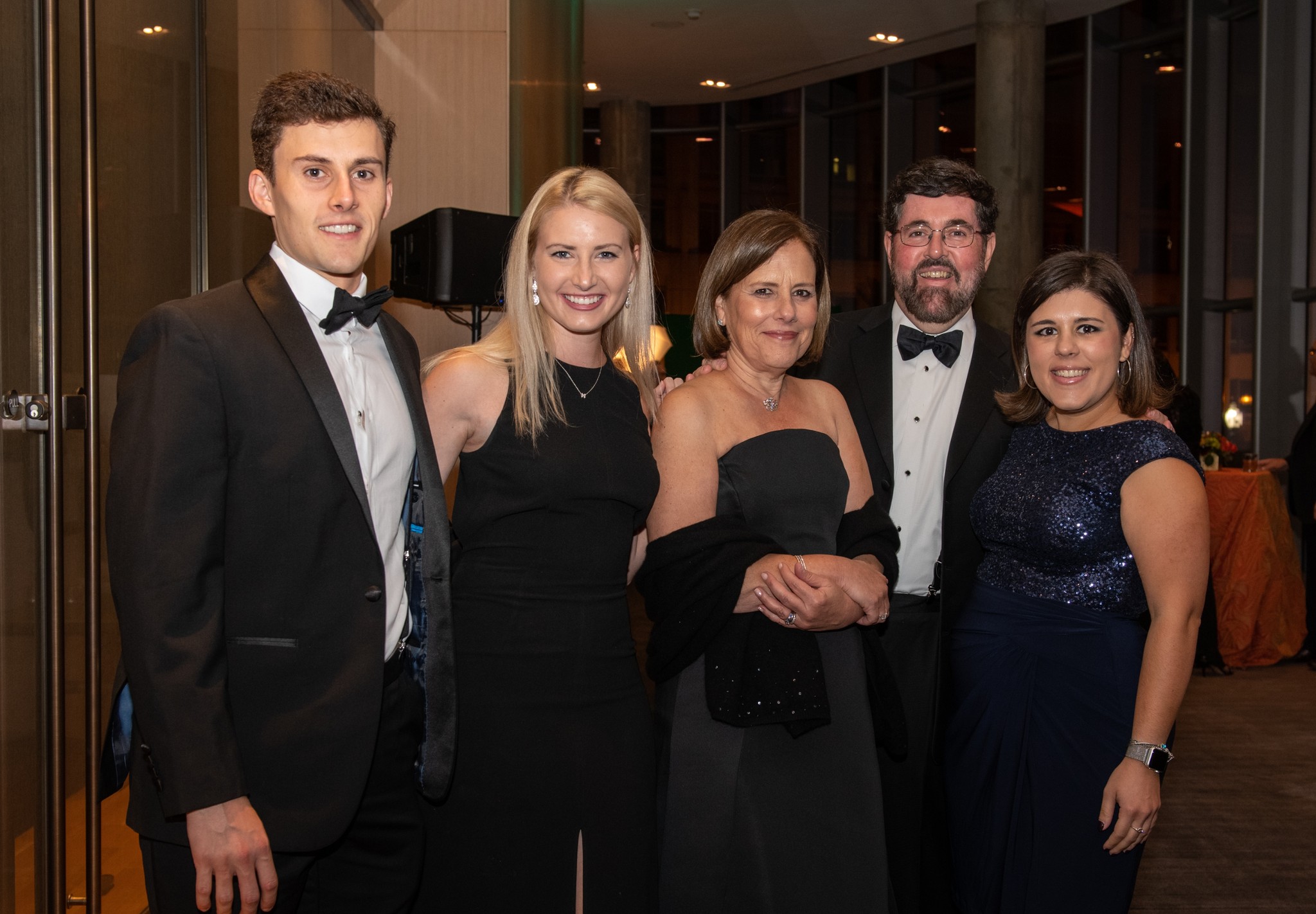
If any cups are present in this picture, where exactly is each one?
[1243,453,1258,472]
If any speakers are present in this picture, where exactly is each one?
[389,207,520,306]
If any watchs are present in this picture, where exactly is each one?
[1125,742,1169,774]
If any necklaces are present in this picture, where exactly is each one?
[548,344,602,400]
[727,364,783,413]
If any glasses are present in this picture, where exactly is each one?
[891,224,992,248]
[1309,346,1316,355]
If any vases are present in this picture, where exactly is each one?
[1199,451,1220,472]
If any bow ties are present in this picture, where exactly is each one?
[318,284,395,335]
[897,324,963,369]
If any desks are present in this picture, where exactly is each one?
[1197,465,1307,667]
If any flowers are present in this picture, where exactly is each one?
[1199,430,1238,461]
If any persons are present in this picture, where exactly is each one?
[935,251,1211,914]
[1135,336,1316,679]
[104,63,469,914]
[418,162,684,913]
[642,207,902,911]
[685,155,1177,914]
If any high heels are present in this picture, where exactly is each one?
[1200,652,1234,676]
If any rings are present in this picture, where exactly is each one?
[784,611,796,626]
[1130,825,1143,834]
[879,610,887,621]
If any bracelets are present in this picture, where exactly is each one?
[1128,739,1175,762]
[794,555,807,572]
